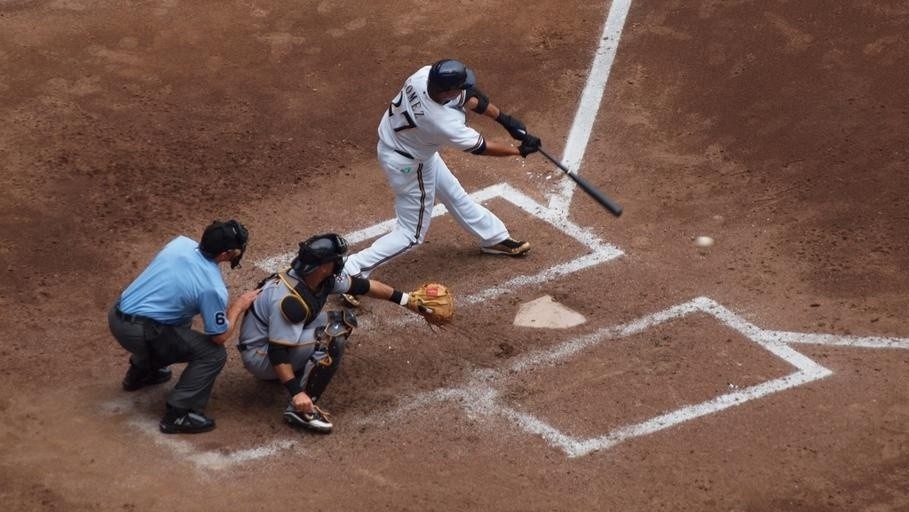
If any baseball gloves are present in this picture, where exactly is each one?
[408,284,454,334]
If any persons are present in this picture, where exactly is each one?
[334,59,542,305]
[108,218,261,434]
[238,233,455,434]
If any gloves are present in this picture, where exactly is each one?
[495,111,542,158]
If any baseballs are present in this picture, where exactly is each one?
[694,236,714,248]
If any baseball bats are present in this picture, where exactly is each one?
[537,145,622,217]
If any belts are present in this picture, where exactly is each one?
[114,307,152,325]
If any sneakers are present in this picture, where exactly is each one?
[338,291,361,307]
[481,237,530,256]
[284,401,334,433]
[122,363,173,392]
[160,409,215,433]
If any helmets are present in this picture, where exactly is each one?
[199,219,249,269]
[428,58,476,95]
[291,233,349,277]
